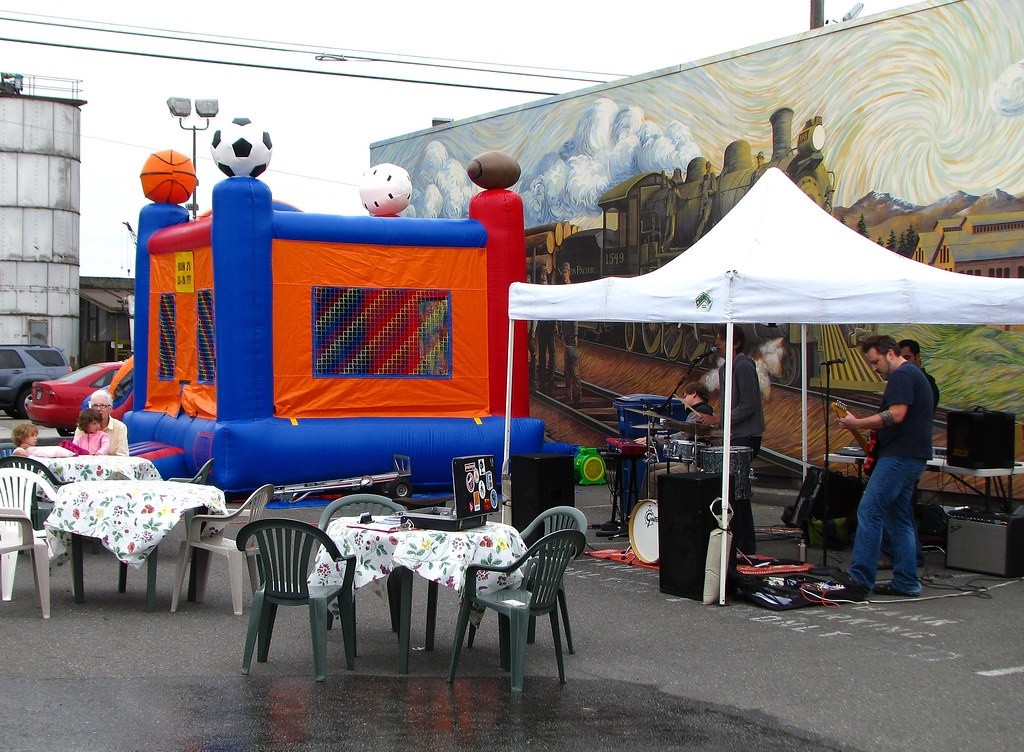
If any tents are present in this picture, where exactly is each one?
[501,167,1024,607]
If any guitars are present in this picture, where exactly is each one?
[831,399,879,476]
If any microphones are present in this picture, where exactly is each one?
[820,357,846,365]
[696,347,716,359]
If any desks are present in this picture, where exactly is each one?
[30,454,164,555]
[44,481,227,613]
[823,446,1024,514]
[307,513,533,675]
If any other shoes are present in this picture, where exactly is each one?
[833,572,864,592]
[872,582,920,598]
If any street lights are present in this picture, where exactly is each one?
[167,96,220,221]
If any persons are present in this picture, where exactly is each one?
[72,389,129,457]
[698,323,766,462]
[11,423,38,456]
[634,382,714,499]
[824,335,934,598]
[897,339,940,416]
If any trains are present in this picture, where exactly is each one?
[555,106,836,387]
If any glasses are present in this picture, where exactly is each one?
[91,402,111,409]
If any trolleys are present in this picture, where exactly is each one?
[271,454,412,505]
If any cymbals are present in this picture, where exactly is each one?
[625,408,674,421]
[662,420,732,440]
[633,424,666,429]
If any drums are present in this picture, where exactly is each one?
[662,439,706,464]
[628,498,659,564]
[698,446,753,500]
[650,431,671,461]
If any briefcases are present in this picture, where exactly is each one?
[946,406,1015,469]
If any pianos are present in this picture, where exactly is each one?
[591,438,647,537]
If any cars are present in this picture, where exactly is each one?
[27,361,124,437]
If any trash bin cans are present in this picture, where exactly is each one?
[613,394,687,514]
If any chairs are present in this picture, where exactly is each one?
[1,441,587,694]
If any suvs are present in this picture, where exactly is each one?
[0,344,73,419]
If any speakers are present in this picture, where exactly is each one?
[509,454,576,552]
[945,410,1015,471]
[658,471,735,601]
[944,507,1024,578]
